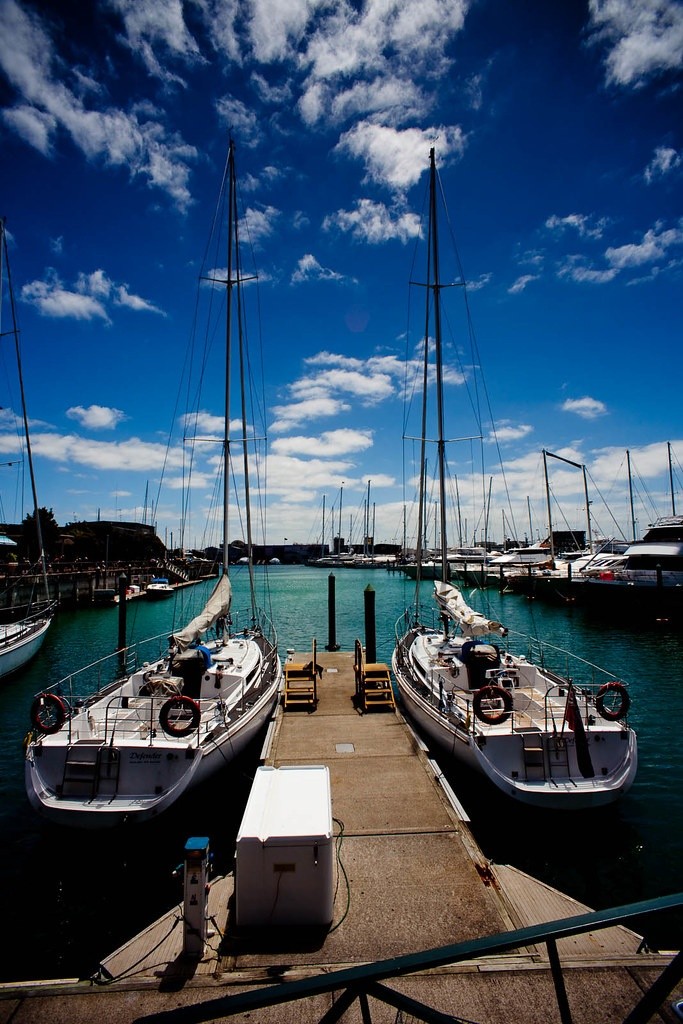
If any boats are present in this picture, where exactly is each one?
[112,585,146,604]
[145,577,174,596]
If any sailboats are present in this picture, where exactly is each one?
[22,126,282,818]
[308,452,511,585]
[481,442,683,591]
[391,146,638,806]
[0,215,55,678]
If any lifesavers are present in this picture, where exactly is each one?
[473,685,513,726]
[595,682,630,721]
[159,696,201,738]
[30,693,66,735]
